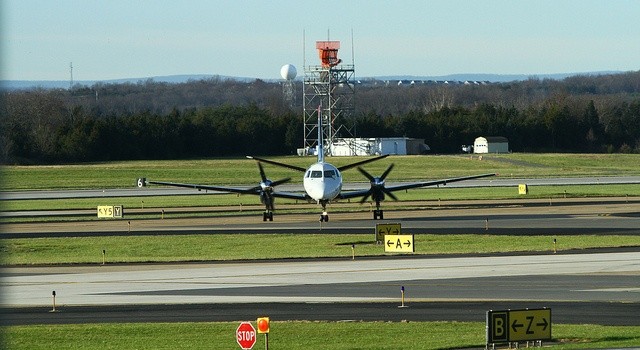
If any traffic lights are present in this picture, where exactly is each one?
[257,317,269,333]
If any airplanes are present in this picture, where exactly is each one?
[144,106,499,222]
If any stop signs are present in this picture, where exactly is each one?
[236,323,257,350]
